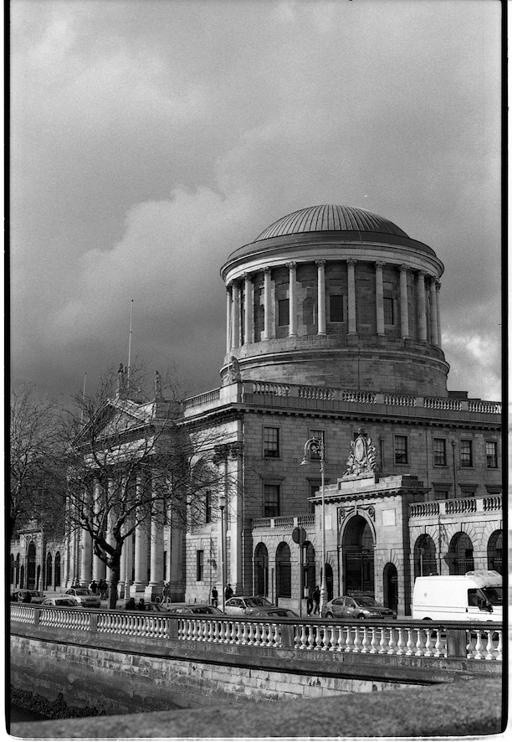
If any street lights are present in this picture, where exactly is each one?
[300,434,328,617]
[218,495,229,613]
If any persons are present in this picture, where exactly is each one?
[306,584,313,616]
[136,598,146,611]
[224,583,233,601]
[210,586,218,607]
[89,578,109,601]
[312,585,321,615]
[125,597,136,610]
